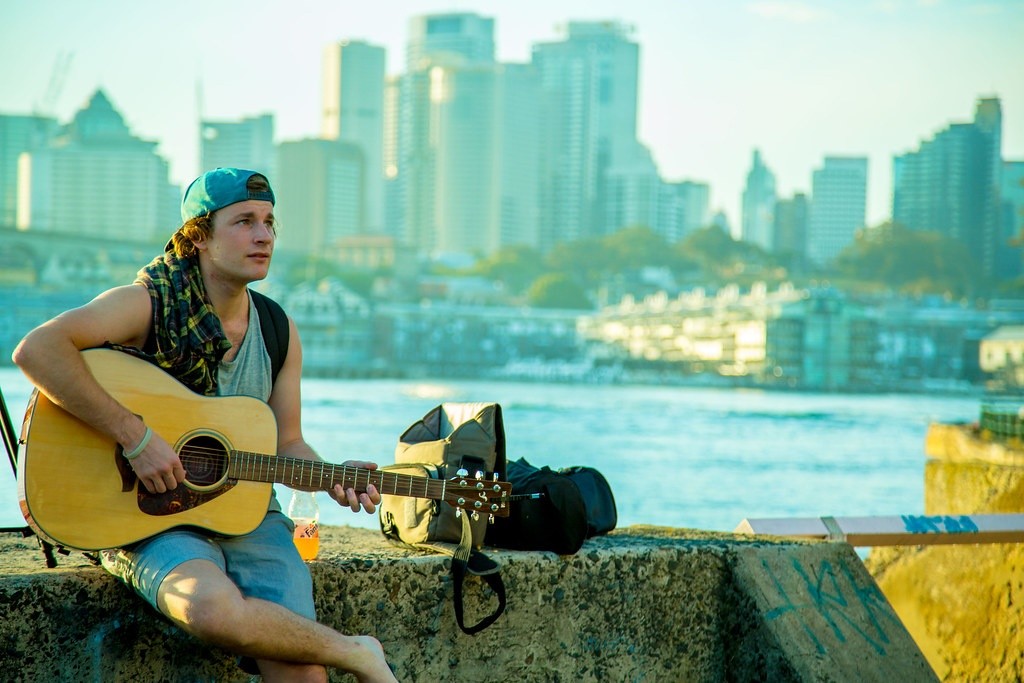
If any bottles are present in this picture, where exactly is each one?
[288,490,320,560]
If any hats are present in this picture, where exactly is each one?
[164,167,275,253]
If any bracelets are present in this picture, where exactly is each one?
[122,426,152,459]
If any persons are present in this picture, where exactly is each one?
[10,167,402,683]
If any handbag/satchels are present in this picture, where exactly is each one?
[378,402,506,634]
[483,454,617,555]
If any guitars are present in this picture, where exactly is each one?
[15,345,514,551]
